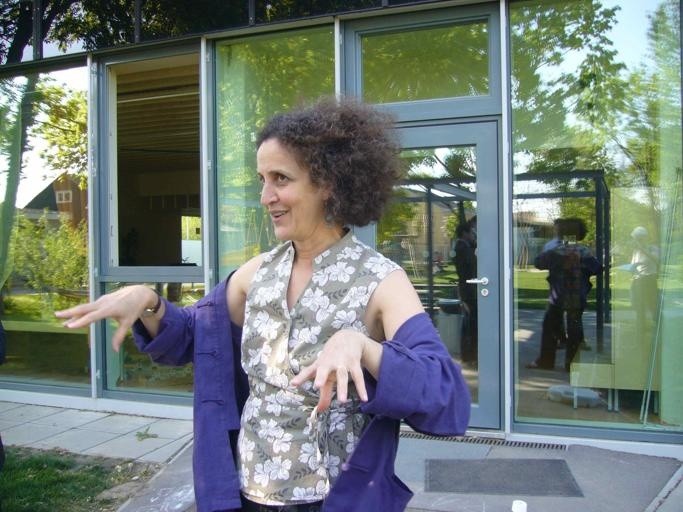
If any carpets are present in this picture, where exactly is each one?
[424,458,585,498]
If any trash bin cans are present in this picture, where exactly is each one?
[436,298,465,356]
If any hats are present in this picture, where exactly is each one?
[631,227,649,239]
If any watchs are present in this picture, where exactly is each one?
[142,287,161,318]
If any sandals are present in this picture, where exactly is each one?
[525,361,538,369]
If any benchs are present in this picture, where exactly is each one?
[570,349,613,412]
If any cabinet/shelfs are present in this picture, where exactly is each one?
[608,187,662,414]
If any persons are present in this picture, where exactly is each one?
[450,214,662,371]
[54,94,471,511]
